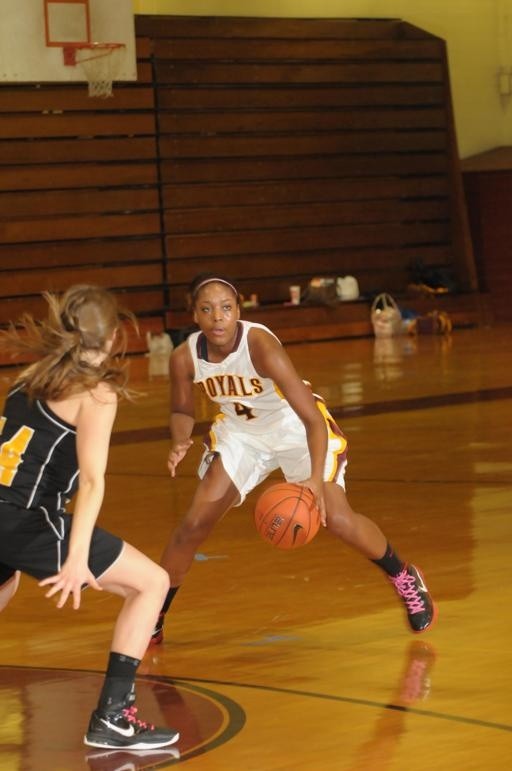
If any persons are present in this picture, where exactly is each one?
[145,269,439,645]
[0,282,181,749]
[86,747,181,771]
[142,639,437,771]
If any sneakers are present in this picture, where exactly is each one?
[82,692,180,750]
[385,560,438,636]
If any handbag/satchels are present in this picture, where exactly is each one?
[370,293,403,338]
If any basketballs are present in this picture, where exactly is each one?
[255,484,320,549]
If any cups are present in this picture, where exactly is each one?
[289,285,301,305]
